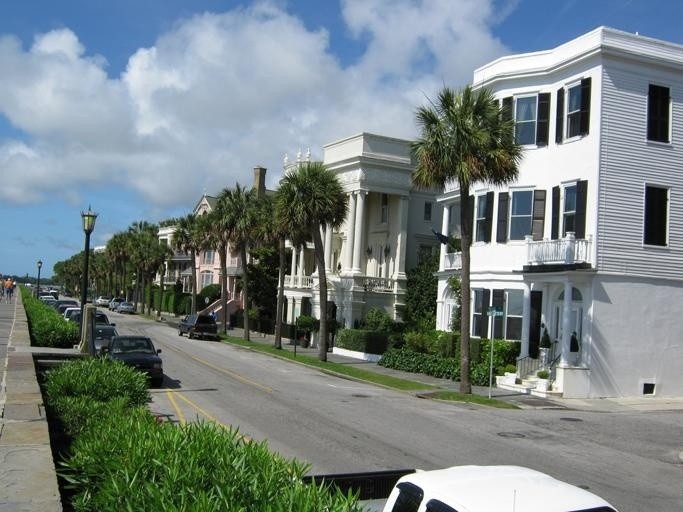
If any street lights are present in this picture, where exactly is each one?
[77,205,99,334]
[34,259,43,299]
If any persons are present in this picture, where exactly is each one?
[5,277,13,304]
[0,273,4,302]
[209,309,219,322]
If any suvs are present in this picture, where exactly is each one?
[178,313,218,341]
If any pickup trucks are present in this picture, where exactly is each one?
[303,460,618,512]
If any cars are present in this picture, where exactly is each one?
[25,283,165,392]
